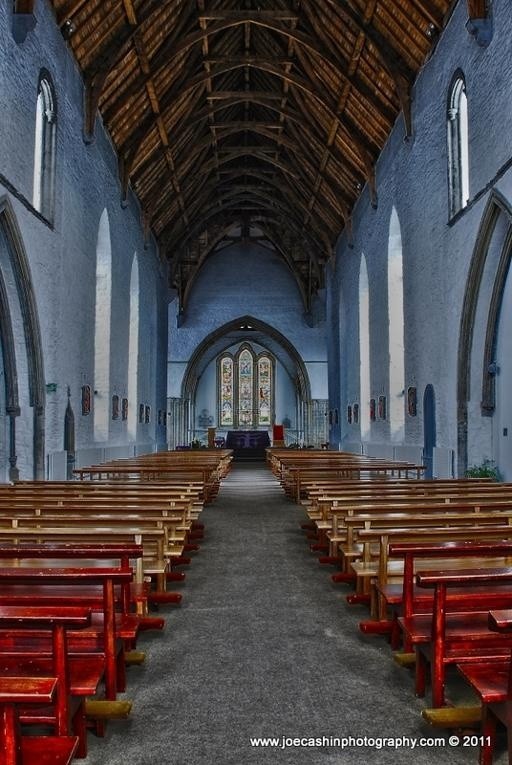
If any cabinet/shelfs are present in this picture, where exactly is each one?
[224,430,270,457]
